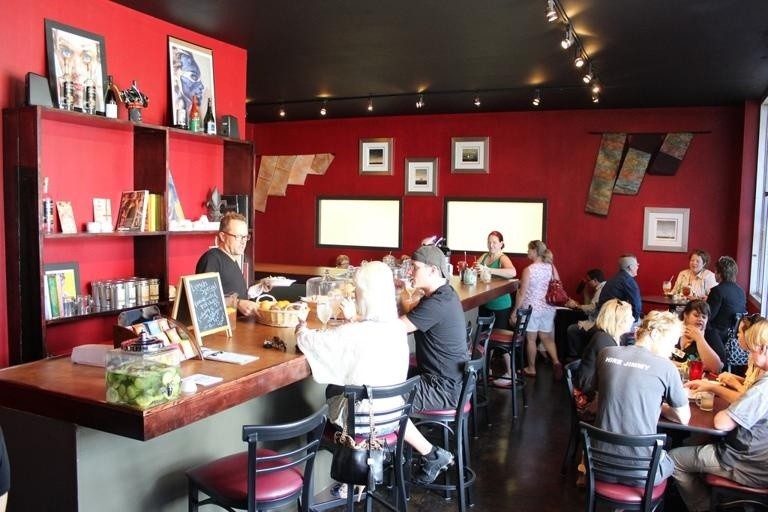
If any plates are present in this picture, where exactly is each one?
[264,279,297,287]
[682,380,720,399]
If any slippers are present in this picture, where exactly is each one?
[553,362,562,381]
[517,368,537,379]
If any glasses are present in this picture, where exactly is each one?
[628,263,640,268]
[221,230,251,241]
[748,312,761,327]
[613,300,623,332]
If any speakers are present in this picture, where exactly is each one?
[25,72,53,107]
[220,115,240,138]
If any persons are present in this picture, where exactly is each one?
[294,260,411,502]
[575,298,635,405]
[395,245,471,484]
[704,257,746,344]
[595,254,642,322]
[565,268,608,365]
[509,240,564,382]
[667,318,768,512]
[718,313,763,394]
[456,230,519,388]
[670,300,726,373]
[194,212,273,319]
[671,250,720,300]
[576,310,692,512]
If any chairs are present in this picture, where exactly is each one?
[479,304,535,420]
[701,475,768,512]
[400,356,490,511]
[182,403,331,512]
[557,356,589,478]
[574,418,668,512]
[470,312,498,360]
[325,373,423,511]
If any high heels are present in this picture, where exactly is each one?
[330,481,366,502]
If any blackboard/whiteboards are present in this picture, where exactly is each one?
[170,275,195,331]
[183,271,233,346]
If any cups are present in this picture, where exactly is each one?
[687,360,704,381]
[480,269,491,284]
[224,292,239,331]
[317,298,332,332]
[101,221,113,233]
[681,284,691,303]
[662,281,672,299]
[458,261,467,282]
[328,294,343,327]
[87,222,101,234]
[106,104,117,118]
[695,392,715,411]
[77,295,92,316]
[63,295,76,317]
[404,281,416,303]
[473,262,480,279]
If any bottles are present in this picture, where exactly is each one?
[60,56,75,112]
[91,281,110,313]
[176,94,187,128]
[110,280,125,311]
[42,176,55,235]
[148,278,160,304]
[204,98,216,134]
[105,76,117,119]
[137,278,149,307]
[124,279,137,309]
[82,63,96,113]
[130,81,141,121]
[190,96,201,132]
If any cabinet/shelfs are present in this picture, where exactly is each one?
[170,125,258,322]
[359,139,394,175]
[2,106,169,366]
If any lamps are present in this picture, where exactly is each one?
[544,0,560,26]
[414,93,426,110]
[277,108,286,119]
[531,92,543,108]
[558,26,579,49]
[365,95,377,112]
[579,67,596,83]
[573,49,590,69]
[319,103,327,118]
[589,80,604,97]
[472,87,482,107]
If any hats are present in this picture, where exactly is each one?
[411,244,455,281]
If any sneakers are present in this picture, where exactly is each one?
[411,445,457,485]
[492,373,518,387]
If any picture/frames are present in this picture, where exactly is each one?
[641,207,691,253]
[165,31,220,132]
[43,20,111,117]
[403,157,440,198]
[452,137,490,174]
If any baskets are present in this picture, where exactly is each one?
[253,294,311,328]
